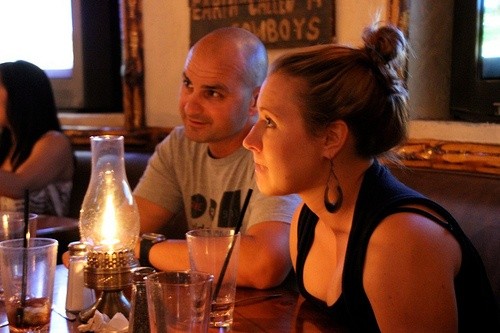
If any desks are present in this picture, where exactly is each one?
[0,262,329,333]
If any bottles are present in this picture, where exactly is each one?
[65,241,96,320]
[128,266,156,333]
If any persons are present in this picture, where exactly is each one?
[62,27,301,290]
[242,28,500,333]
[0,61,74,218]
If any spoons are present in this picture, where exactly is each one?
[52,303,76,321]
[212,294,283,311]
[0,321,9,327]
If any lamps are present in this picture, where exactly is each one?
[78,136,140,325]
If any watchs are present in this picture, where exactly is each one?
[138,232,166,272]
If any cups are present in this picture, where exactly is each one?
[0,212,38,300]
[0,238,59,333]
[186,228,241,316]
[143,271,214,333]
[208,316,233,333]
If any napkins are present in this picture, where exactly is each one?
[77,309,129,333]
